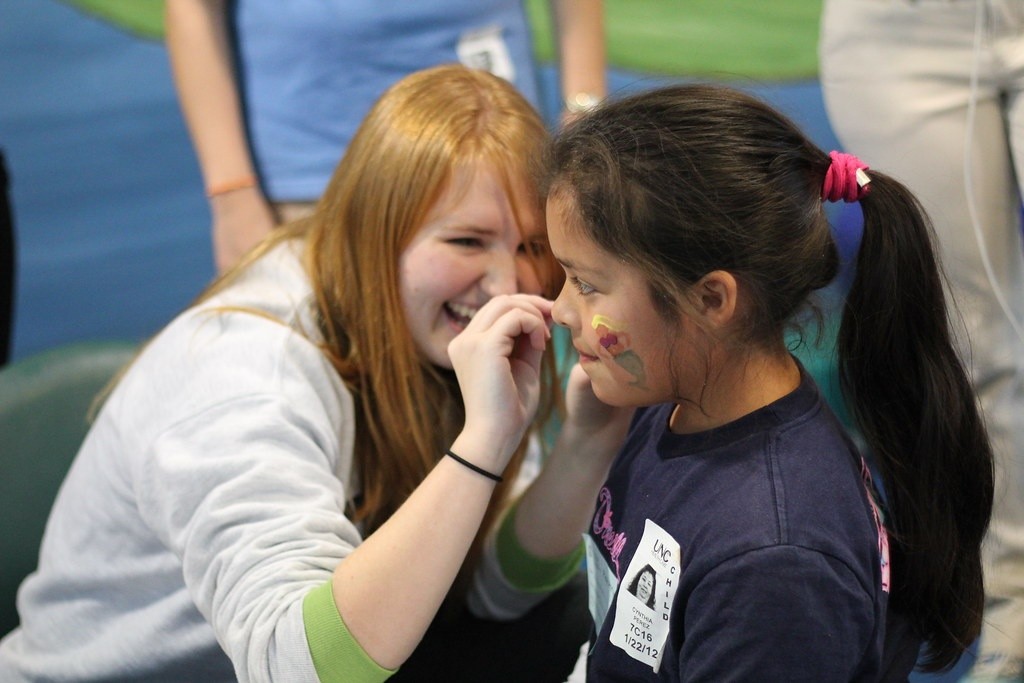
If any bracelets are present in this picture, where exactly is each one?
[205,173,258,199]
[560,95,604,114]
[446,450,503,484]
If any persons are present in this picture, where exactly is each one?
[539,77,997,683]
[3,64,637,683]
[156,0,613,283]
[813,0,1023,683]
